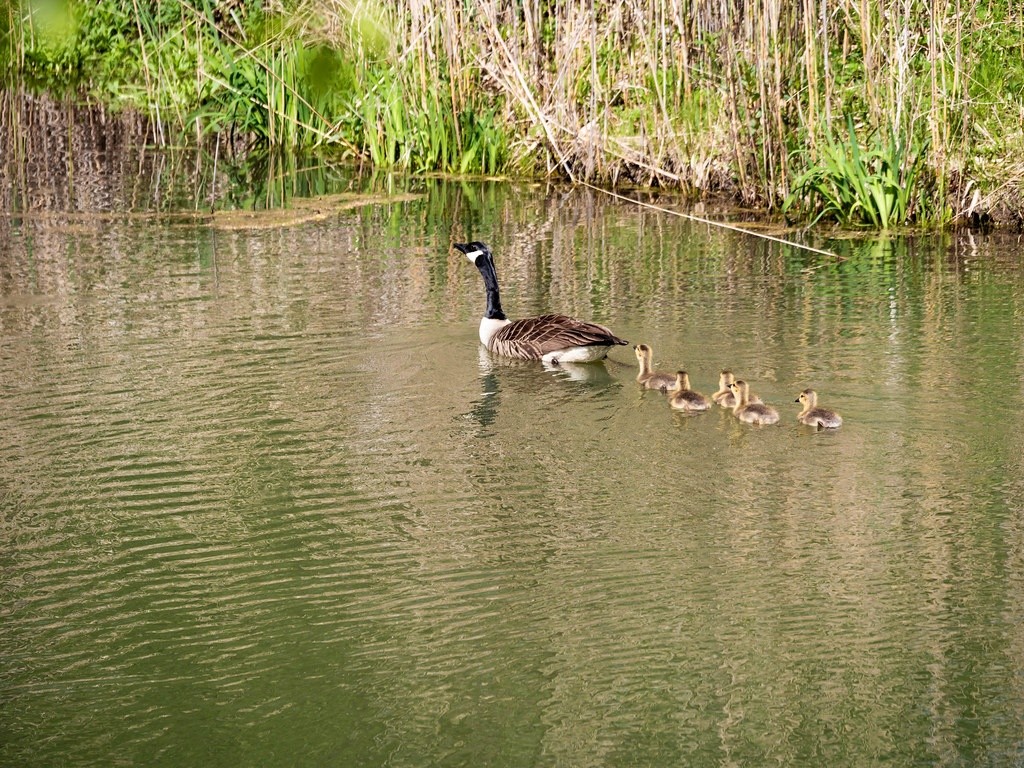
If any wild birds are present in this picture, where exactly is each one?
[632,344,676,391]
[453,241,629,363]
[710,370,761,408]
[795,388,843,428]
[668,370,712,410]
[724,380,779,425]
